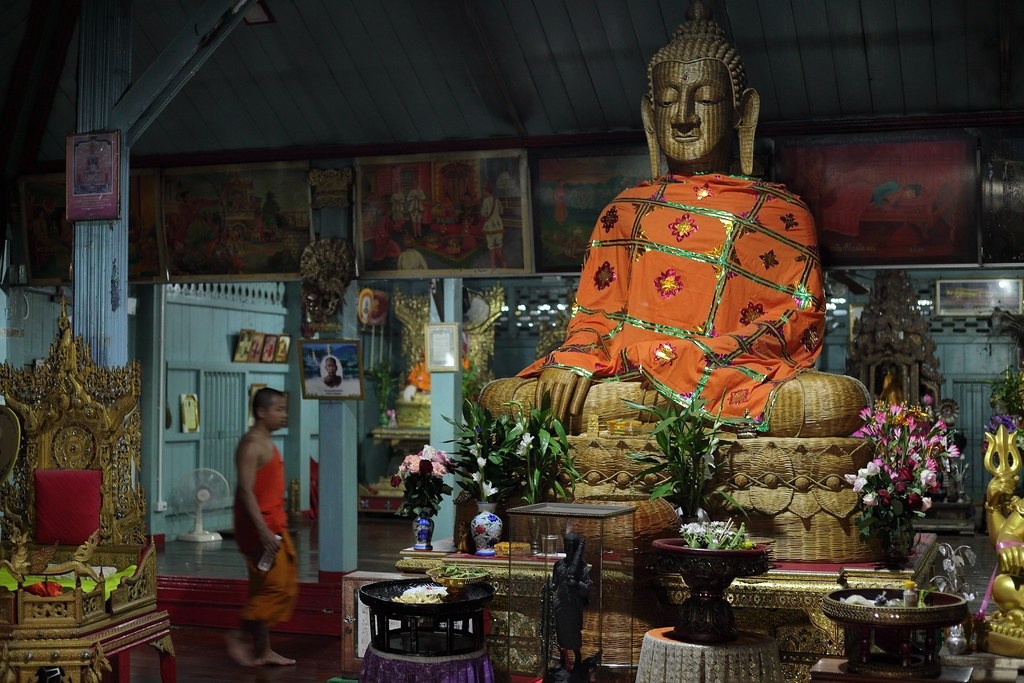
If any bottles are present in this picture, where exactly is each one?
[903,581,918,607]
[257,535,282,572]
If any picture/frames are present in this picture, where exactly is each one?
[350,148,533,277]
[14,165,166,285]
[933,275,1023,317]
[245,383,267,429]
[160,159,316,282]
[768,125,978,264]
[231,328,293,364]
[179,394,200,433]
[295,337,365,401]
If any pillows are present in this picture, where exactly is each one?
[30,469,102,546]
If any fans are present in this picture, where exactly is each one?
[174,468,229,542]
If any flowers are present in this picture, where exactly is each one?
[498,401,586,506]
[677,516,758,551]
[439,397,526,503]
[387,444,456,516]
[850,394,959,541]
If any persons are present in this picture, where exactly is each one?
[323,358,341,386]
[984,472,1024,627]
[542,532,591,675]
[477,0,872,437]
[226,387,298,665]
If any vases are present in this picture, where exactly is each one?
[652,539,767,646]
[873,505,913,571]
[411,510,433,550]
[526,514,540,556]
[470,500,502,557]
